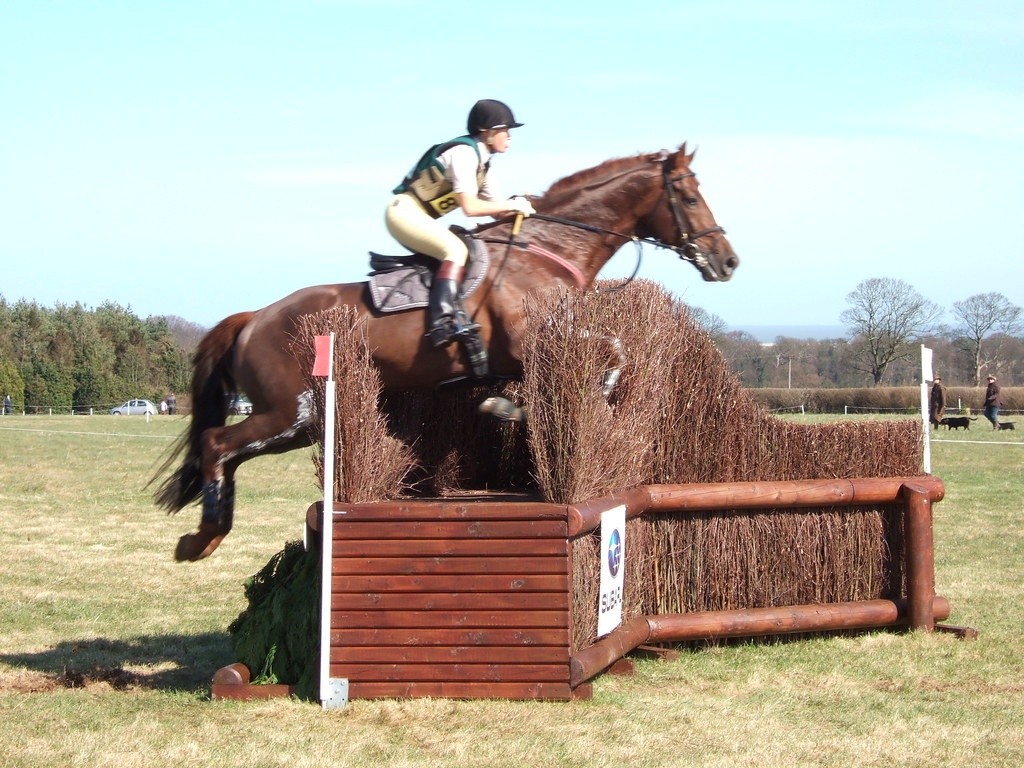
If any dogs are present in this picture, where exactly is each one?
[939,417,978,432]
[998,422,1016,431]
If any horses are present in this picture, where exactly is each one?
[140,140,739,562]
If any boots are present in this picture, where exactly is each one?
[430,261,480,348]
[993,421,1000,430]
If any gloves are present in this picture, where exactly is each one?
[507,196,538,218]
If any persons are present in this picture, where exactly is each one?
[166,393,177,415]
[930,376,946,430]
[984,374,1000,431]
[385,99,537,348]
[5,394,13,415]
[159,400,167,415]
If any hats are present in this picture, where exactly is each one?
[933,374,941,379]
[467,100,523,137]
[987,374,996,380]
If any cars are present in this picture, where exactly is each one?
[224,393,253,415]
[109,400,158,415]
